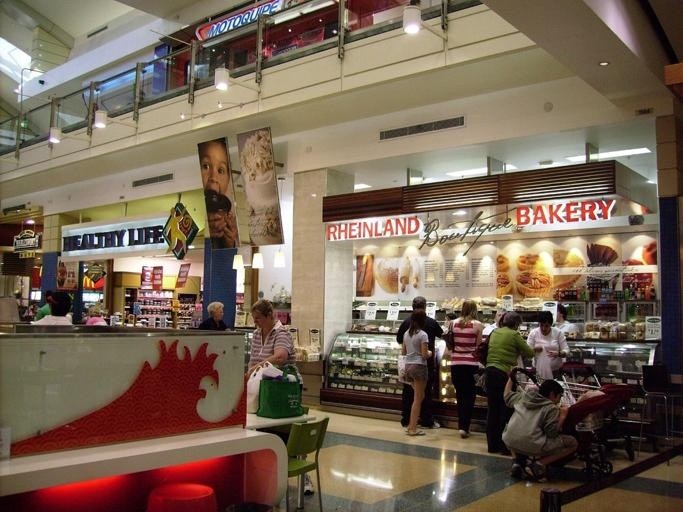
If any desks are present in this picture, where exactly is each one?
[0,429,288,512]
[245,413,316,510]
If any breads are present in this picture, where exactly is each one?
[496,252,507,271]
[375,259,398,294]
[642,241,656,265]
[553,250,584,289]
[356,256,374,297]
[516,253,540,271]
[515,270,549,298]
[496,273,513,298]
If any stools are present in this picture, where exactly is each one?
[148,482,218,512]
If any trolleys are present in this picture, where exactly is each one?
[510,360,639,472]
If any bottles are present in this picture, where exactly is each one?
[553,275,656,300]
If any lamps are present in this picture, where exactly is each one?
[232,244,286,269]
[402,0,423,34]
[94,81,107,129]
[214,32,230,92]
[49,99,62,143]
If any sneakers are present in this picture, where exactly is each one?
[303,474,314,494]
[511,463,526,478]
[420,421,440,429]
[526,461,548,483]
[459,429,466,437]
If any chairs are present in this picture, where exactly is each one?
[637,365,683,466]
[287,417,329,511]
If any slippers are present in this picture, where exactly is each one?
[407,430,425,436]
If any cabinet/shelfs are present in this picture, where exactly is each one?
[123,287,197,329]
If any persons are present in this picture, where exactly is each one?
[527,312,570,380]
[397,297,446,429]
[440,313,457,334]
[502,369,578,481]
[447,300,483,438]
[482,308,506,341]
[198,302,234,330]
[198,140,236,249]
[18,293,105,324]
[400,308,432,437]
[484,312,535,453]
[557,303,581,334]
[247,299,318,494]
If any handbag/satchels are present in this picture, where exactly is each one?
[256,378,303,419]
[472,342,487,367]
[398,355,411,385]
[444,331,454,349]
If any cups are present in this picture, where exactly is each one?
[206,193,232,249]
